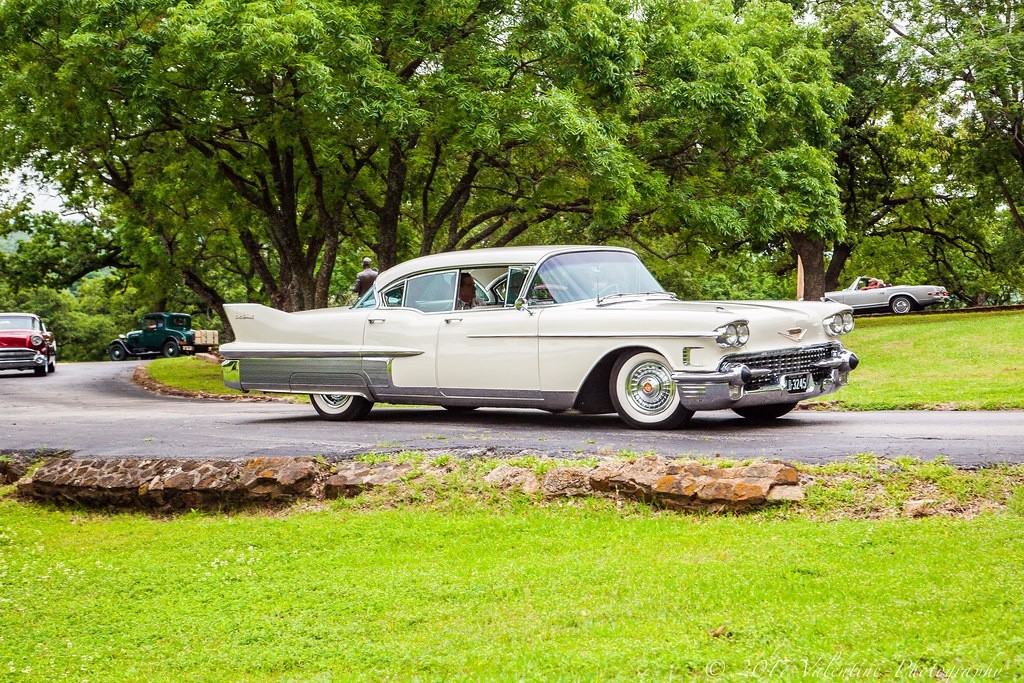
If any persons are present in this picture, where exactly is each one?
[451,272,486,311]
[350,257,378,300]
[506,272,529,305]
[149,320,164,329]
[860,278,884,290]
[176,318,183,325]
[557,267,599,303]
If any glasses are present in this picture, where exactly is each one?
[460,282,477,288]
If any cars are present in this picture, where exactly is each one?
[109,312,208,360]
[825,274,951,316]
[217,242,859,432]
[0,311,58,374]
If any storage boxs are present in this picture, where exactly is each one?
[196,330,218,344]
